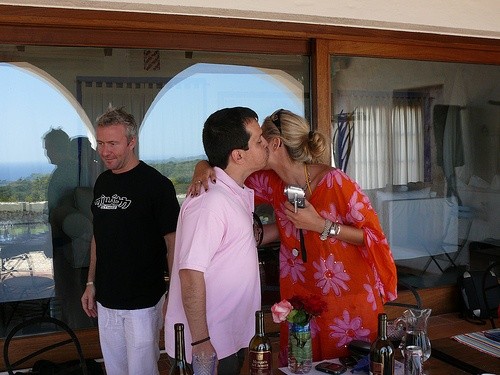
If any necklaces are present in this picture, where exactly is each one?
[303,172,311,191]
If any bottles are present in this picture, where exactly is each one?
[249,310,272,375]
[370,313,395,374]
[170,323,193,375]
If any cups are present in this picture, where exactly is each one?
[193,352,216,375]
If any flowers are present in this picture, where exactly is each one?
[271,296,327,348]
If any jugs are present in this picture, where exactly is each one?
[395,308,432,361]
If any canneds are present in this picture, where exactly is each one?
[404,346,424,375]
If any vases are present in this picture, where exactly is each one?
[287,322,312,374]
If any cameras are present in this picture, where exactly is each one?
[284,185,306,208]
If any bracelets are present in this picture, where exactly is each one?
[86,282,93,285]
[321,221,340,240]
[191,337,210,346]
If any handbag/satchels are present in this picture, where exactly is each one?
[454,271,500,322]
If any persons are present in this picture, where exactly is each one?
[164,105,280,375]
[44,129,99,329]
[81,105,180,375]
[186,108,397,362]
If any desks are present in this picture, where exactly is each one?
[274,328,500,375]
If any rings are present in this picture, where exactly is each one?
[198,181,200,183]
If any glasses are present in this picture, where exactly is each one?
[270,108,283,135]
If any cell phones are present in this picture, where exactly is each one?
[315,361,348,375]
